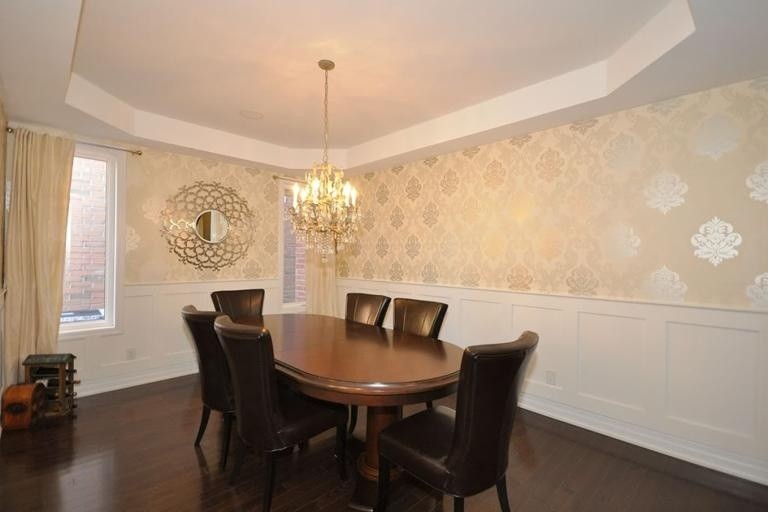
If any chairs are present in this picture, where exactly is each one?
[379,331,541,511]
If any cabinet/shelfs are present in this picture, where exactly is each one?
[22,352,75,421]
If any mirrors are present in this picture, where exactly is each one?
[193,209,231,243]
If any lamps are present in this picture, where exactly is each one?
[287,59,361,255]
